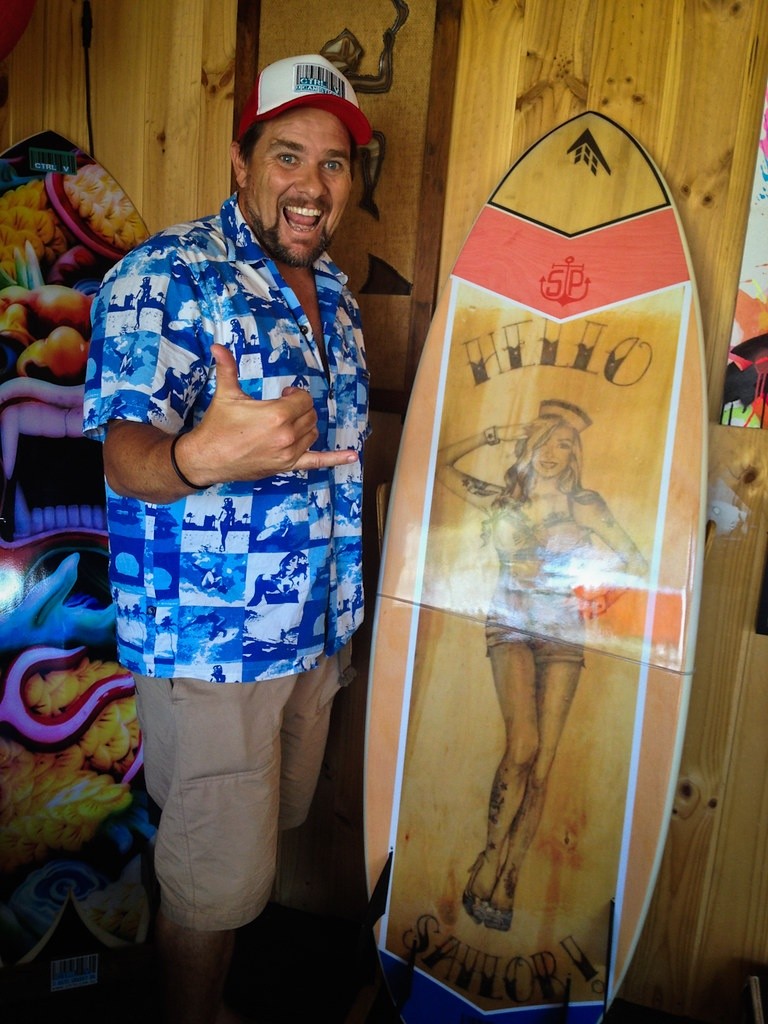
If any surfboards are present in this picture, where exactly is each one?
[0,130,162,1024]
[362,105,713,1023]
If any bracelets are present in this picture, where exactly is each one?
[171,429,214,489]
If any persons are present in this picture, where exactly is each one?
[80,51,373,1024]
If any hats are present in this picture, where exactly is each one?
[237,54,373,148]
